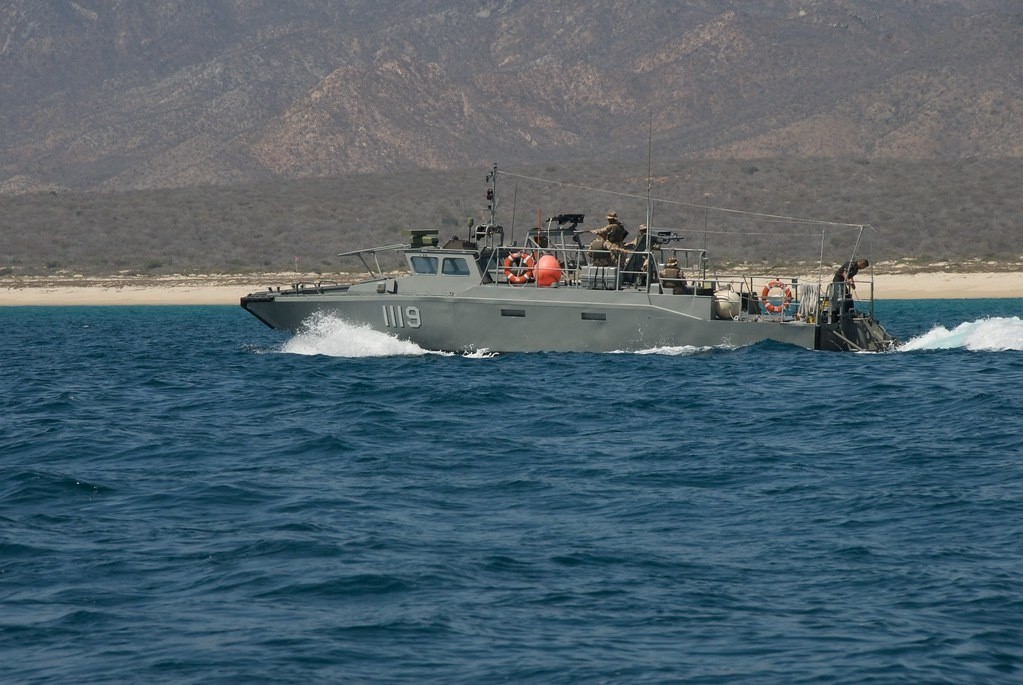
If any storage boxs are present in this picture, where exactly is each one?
[581,266,623,290]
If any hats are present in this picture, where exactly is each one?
[639,224,647,231]
[605,211,621,221]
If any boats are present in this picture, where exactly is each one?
[240,98,908,358]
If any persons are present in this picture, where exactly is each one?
[832,260,869,322]
[587,211,625,268]
[624,224,647,286]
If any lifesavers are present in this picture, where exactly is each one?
[762,281,792,313]
[504,254,534,282]
